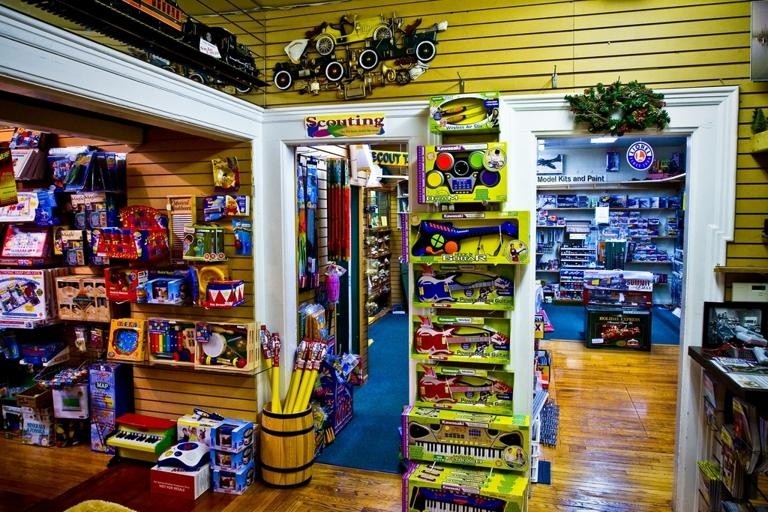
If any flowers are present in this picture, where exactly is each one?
[564,75,671,137]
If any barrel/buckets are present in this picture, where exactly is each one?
[261,401,316,487]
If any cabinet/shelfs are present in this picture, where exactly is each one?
[536,173,686,304]
[688,346,768,512]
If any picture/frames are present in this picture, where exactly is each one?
[703,302,768,349]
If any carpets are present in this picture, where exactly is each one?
[314,314,409,474]
[539,302,680,345]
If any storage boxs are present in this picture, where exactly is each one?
[150,412,260,502]
